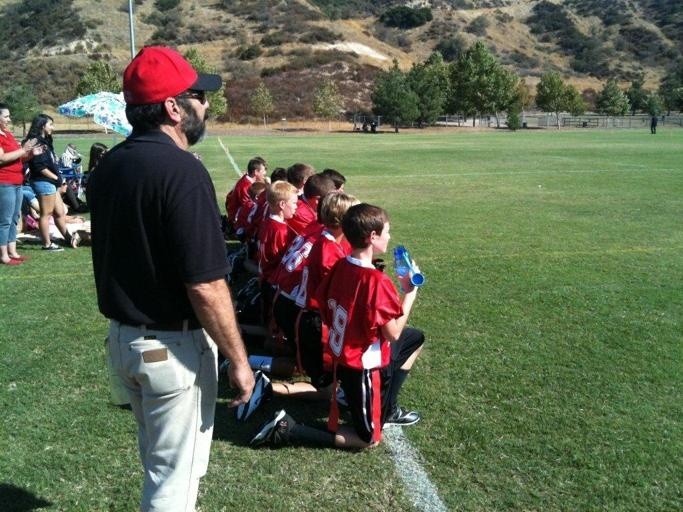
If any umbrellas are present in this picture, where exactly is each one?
[57,90,133,139]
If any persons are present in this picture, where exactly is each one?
[0,106,108,267]
[353,119,377,132]
[220,157,426,448]
[84,45,255,512]
[650,113,658,134]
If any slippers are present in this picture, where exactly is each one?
[42,242,64,251]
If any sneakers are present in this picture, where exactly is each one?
[1,254,29,264]
[236,276,262,319]
[220,358,294,445]
[380,404,421,429]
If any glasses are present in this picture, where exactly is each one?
[179,90,208,104]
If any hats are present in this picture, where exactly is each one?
[123,46,221,103]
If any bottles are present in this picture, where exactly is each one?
[393,245,424,294]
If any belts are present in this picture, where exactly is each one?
[120,319,201,331]
